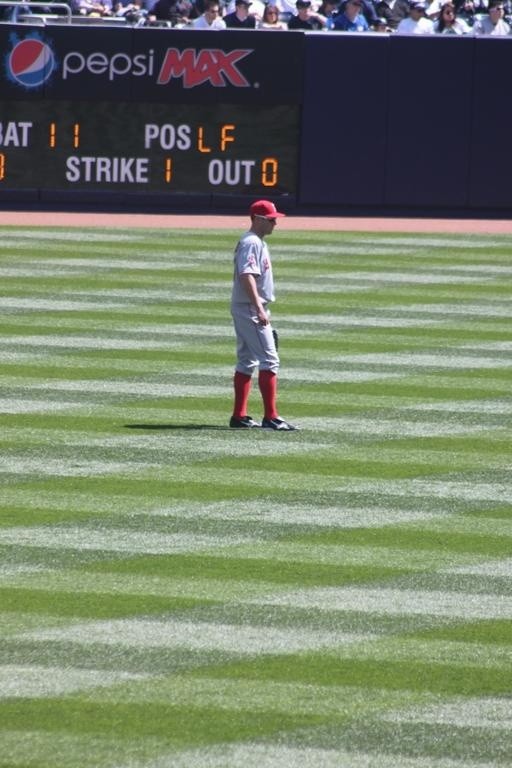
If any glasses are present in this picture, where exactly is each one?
[347,2,361,7]
[496,7,503,10]
[347,0,363,2]
[443,10,453,15]
[205,10,219,13]
[268,12,278,15]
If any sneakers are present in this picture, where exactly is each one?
[229,415,262,429]
[262,416,296,430]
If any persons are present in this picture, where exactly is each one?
[2,0,511,38]
[228,197,299,431]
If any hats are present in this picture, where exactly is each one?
[235,0,253,6]
[296,0,311,6]
[410,2,428,9]
[488,0,508,7]
[251,200,285,219]
